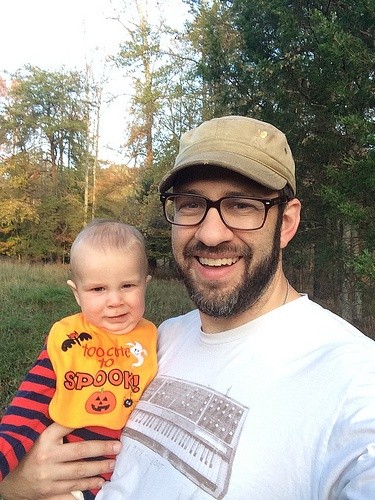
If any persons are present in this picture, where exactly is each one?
[0,219,159,500]
[0,116,374,500]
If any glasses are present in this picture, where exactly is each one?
[161,190,289,231]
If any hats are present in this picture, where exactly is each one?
[158,116,297,197]
